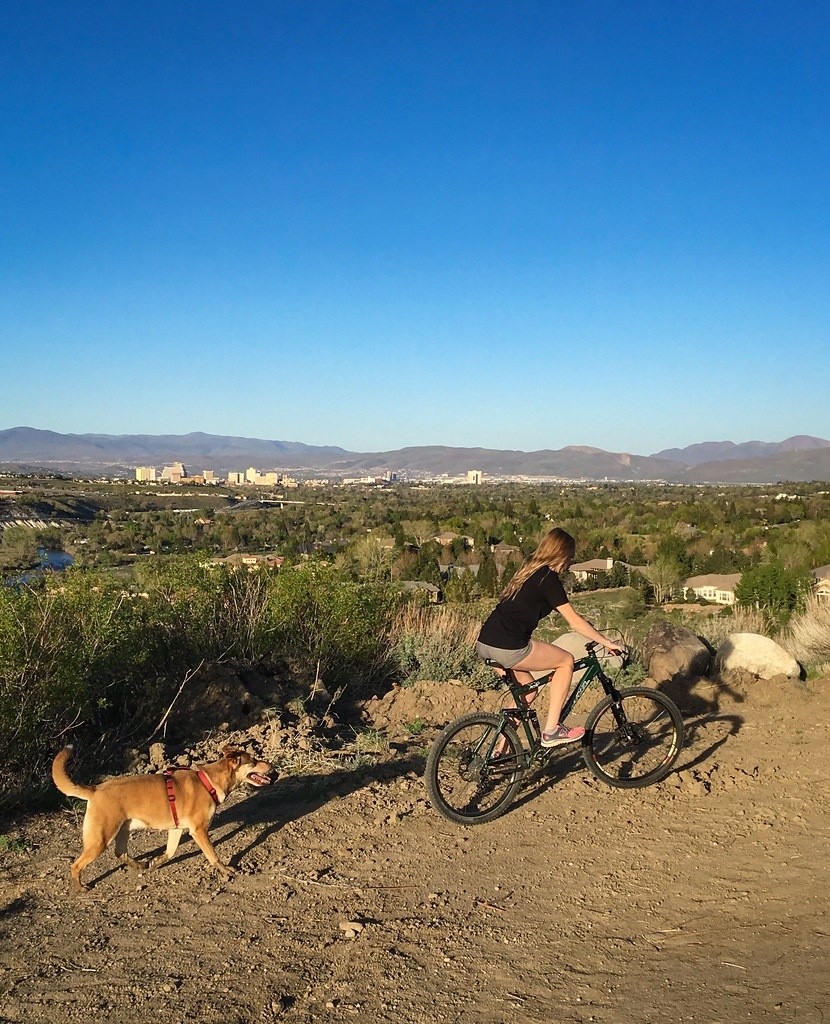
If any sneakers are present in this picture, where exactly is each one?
[491,750,516,766]
[540,722,585,748]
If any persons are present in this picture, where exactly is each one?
[476,528,625,759]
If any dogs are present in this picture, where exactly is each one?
[50,744,273,893]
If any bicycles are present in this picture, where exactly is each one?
[424,621,684,826]
